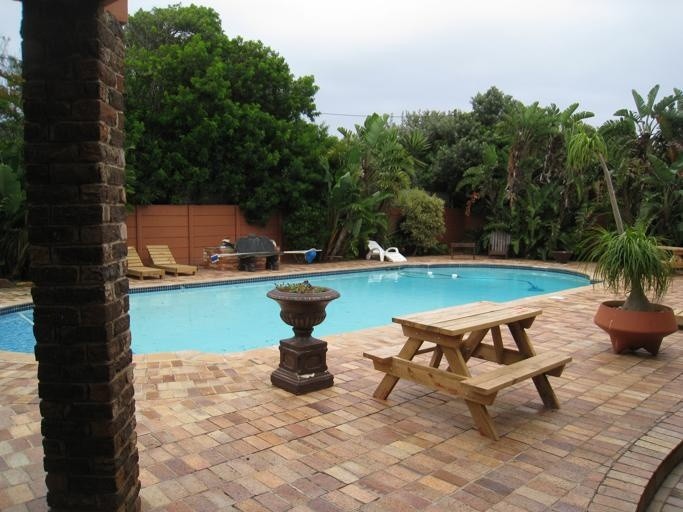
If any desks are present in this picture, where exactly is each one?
[282,249,322,264]
[376,301,561,442]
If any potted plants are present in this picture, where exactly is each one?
[266,280,341,396]
[572,219,680,356]
[480,221,518,260]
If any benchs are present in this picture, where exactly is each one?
[363,335,573,441]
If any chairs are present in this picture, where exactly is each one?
[366,240,407,263]
[125,244,197,282]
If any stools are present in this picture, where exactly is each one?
[449,242,477,260]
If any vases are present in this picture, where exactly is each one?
[549,249,573,263]
